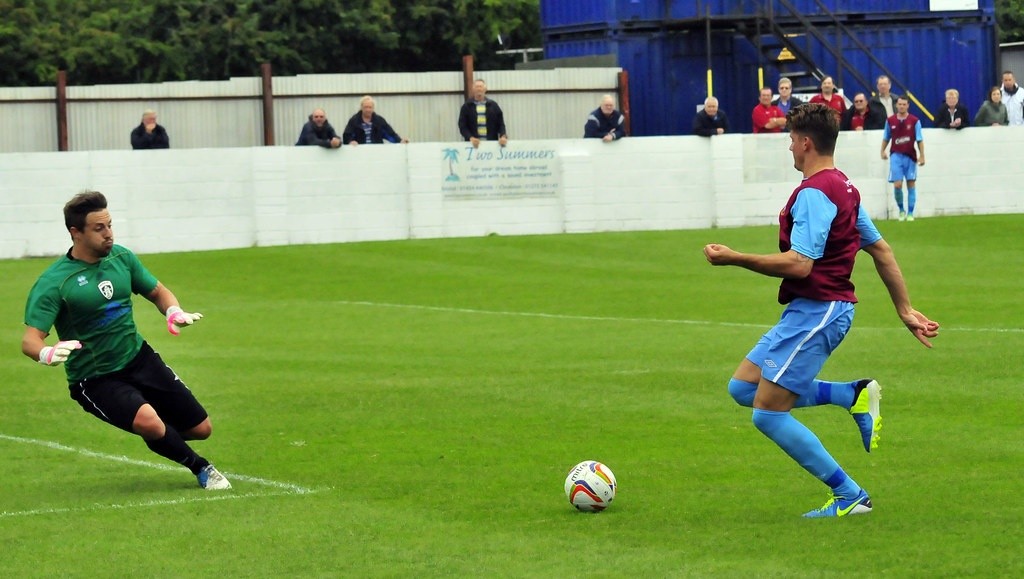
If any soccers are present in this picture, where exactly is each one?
[564,460,616,512]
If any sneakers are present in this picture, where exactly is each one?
[196,463,233,492]
[847,379,884,454]
[801,487,873,521]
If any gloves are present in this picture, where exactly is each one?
[165,305,204,335]
[38,339,82,366]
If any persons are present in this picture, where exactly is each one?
[772,78,804,132]
[584,94,626,141]
[840,92,885,130]
[296,108,342,148]
[973,87,1009,126]
[880,94,924,223]
[130,111,170,149]
[868,75,900,125]
[21,192,232,491]
[704,103,940,519]
[693,96,728,136]
[809,76,846,124]
[999,71,1024,126]
[752,86,788,133]
[343,96,408,145]
[932,88,970,129]
[457,80,507,147]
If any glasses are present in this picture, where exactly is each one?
[855,99,866,104]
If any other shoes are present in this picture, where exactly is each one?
[908,211,915,222]
[898,210,906,223]
